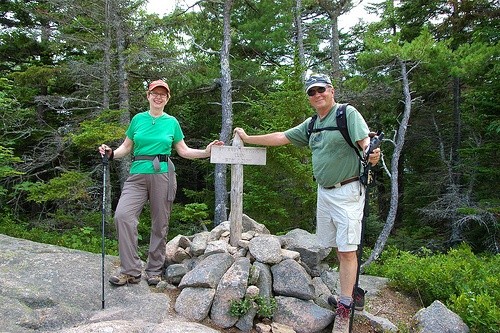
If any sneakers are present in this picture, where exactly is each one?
[332,299,354,333]
[328,286,367,311]
[109,272,143,287]
[148,275,161,285]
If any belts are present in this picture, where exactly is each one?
[325,176,358,189]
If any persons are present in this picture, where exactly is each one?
[233,74,381,333]
[99,79,224,285]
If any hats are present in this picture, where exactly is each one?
[306,74,334,93]
[149,79,170,97]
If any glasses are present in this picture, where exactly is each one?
[307,87,327,96]
[149,91,167,98]
